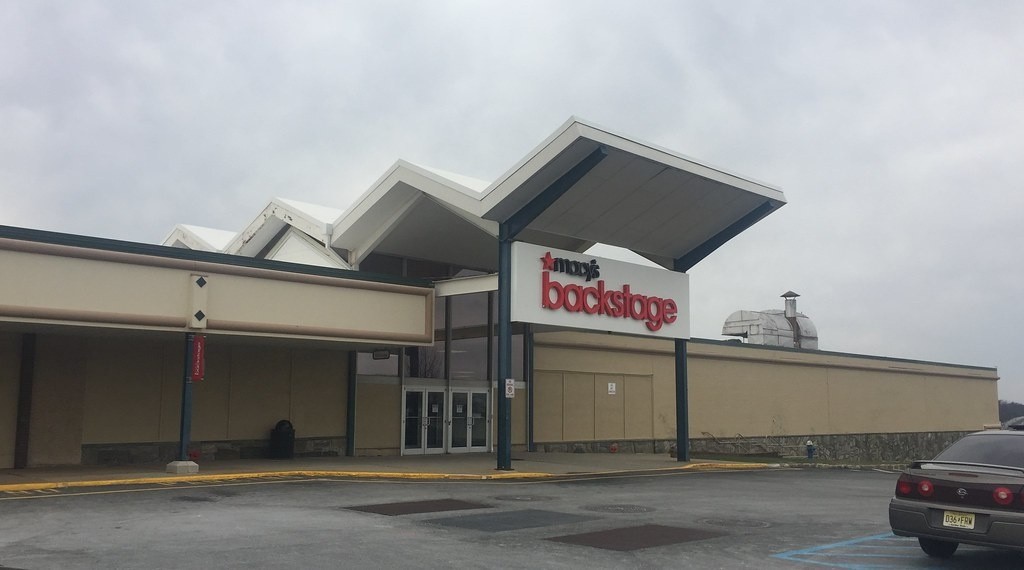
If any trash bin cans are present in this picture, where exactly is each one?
[269,418,295,459]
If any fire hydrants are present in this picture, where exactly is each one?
[806,439,816,460]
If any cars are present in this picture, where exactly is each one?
[888,426,1024,565]
